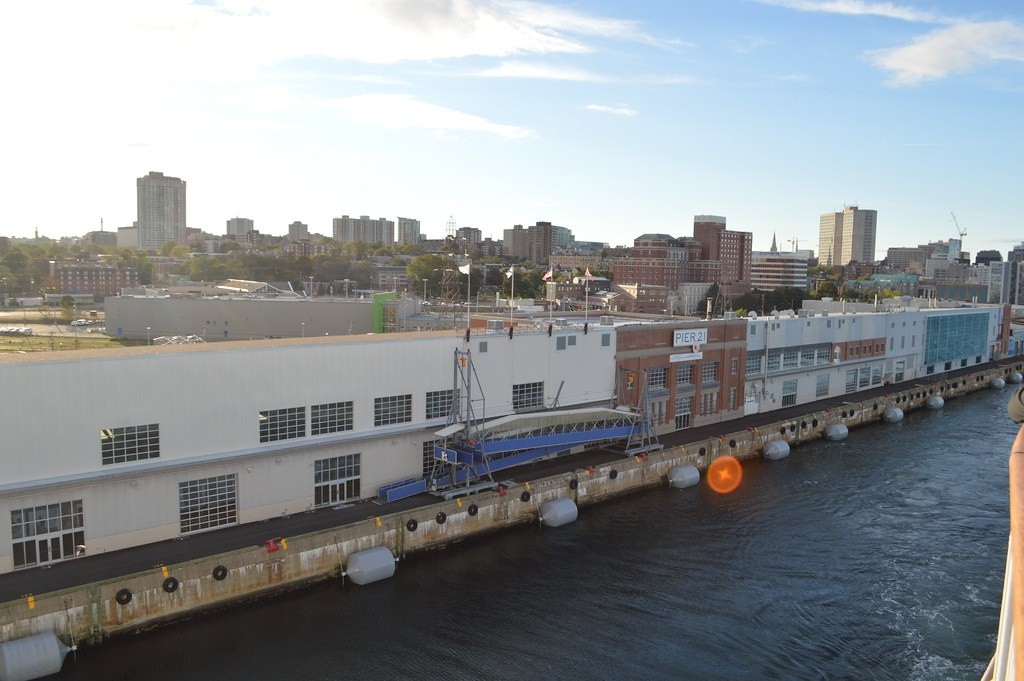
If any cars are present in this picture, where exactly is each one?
[71,319,105,326]
[0,327,32,335]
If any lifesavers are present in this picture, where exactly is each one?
[163,576,179,593]
[521,491,530,502]
[610,469,618,479]
[436,512,446,524]
[841,408,856,418]
[468,504,478,516]
[116,589,134,606]
[730,439,737,447]
[570,479,577,489]
[1005,365,1023,374]
[406,519,418,532]
[699,447,706,456]
[874,403,878,410]
[896,375,985,403]
[213,565,227,581]
[781,419,819,435]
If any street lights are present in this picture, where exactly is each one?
[310,276,398,298]
[422,279,429,302]
[147,327,151,345]
[302,323,305,337]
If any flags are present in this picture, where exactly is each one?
[542,268,552,280]
[458,264,469,274]
[585,267,593,281]
[505,266,512,278]
